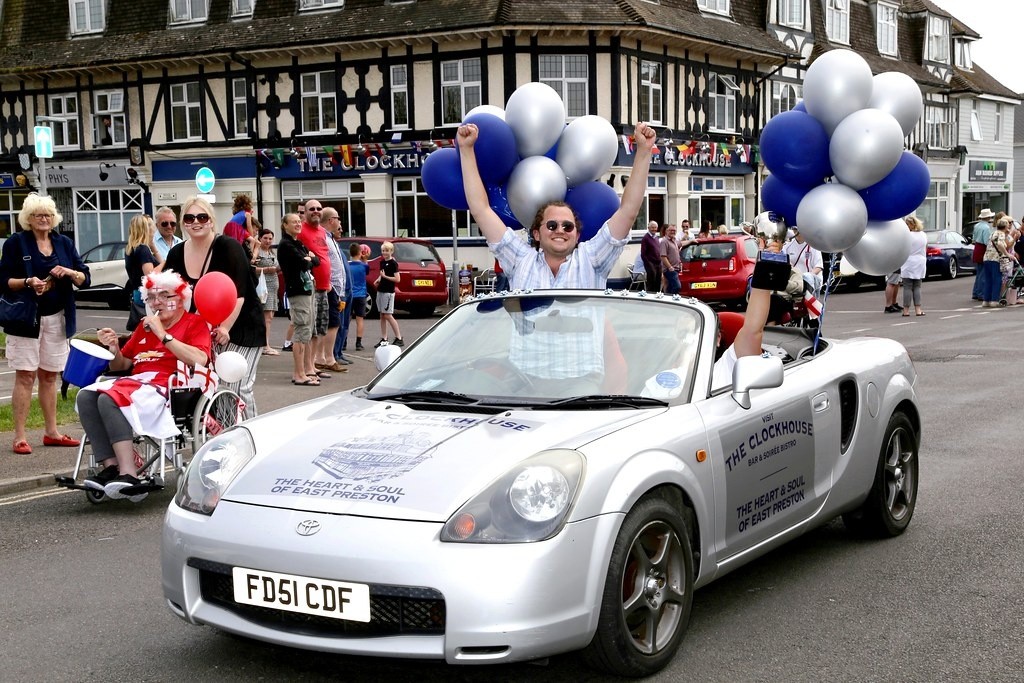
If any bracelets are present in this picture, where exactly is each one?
[23,277,32,289]
[71,270,79,282]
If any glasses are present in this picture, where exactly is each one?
[146,295,177,301]
[30,213,55,219]
[309,207,322,211]
[682,226,688,228]
[297,211,306,214]
[1009,221,1013,224]
[675,330,695,340]
[183,213,212,223]
[329,216,341,221]
[159,221,177,227]
[539,220,577,232]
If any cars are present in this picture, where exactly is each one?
[674,234,758,312]
[71,240,136,311]
[923,229,978,280]
[332,235,448,320]
[961,220,1022,244]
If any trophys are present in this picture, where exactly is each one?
[736,210,800,289]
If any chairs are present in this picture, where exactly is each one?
[708,244,725,257]
[593,308,630,396]
[713,310,748,356]
[472,267,497,297]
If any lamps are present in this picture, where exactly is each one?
[36,164,55,182]
[98,160,116,181]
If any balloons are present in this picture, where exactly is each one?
[420,82,622,244]
[214,351,247,383]
[193,271,238,327]
[757,49,932,277]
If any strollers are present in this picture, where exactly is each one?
[998,255,1023,308]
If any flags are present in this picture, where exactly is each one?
[621,134,762,167]
[257,138,457,170]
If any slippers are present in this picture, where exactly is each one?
[902,313,910,316]
[916,312,926,316]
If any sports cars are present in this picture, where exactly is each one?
[157,283,927,682]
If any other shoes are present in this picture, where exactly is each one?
[43,434,80,446]
[261,337,405,386]
[13,441,32,453]
[999,255,1010,272]
[989,300,1001,307]
[982,300,990,307]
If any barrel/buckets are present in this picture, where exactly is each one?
[63,328,116,388]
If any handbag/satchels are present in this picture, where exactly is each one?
[0,278,39,326]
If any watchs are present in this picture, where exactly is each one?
[160,333,174,346]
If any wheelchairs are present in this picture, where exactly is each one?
[55,329,250,505]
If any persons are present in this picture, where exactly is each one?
[455,118,656,401]
[0,192,92,455]
[640,219,729,295]
[635,239,784,404]
[784,227,824,336]
[75,269,211,503]
[972,207,1024,306]
[124,195,404,387]
[902,216,927,316]
[886,269,903,310]
[158,196,268,431]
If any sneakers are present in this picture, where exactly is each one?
[104,473,149,503]
[885,305,900,313]
[893,303,904,310]
[83,465,120,491]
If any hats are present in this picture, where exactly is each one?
[978,208,995,218]
